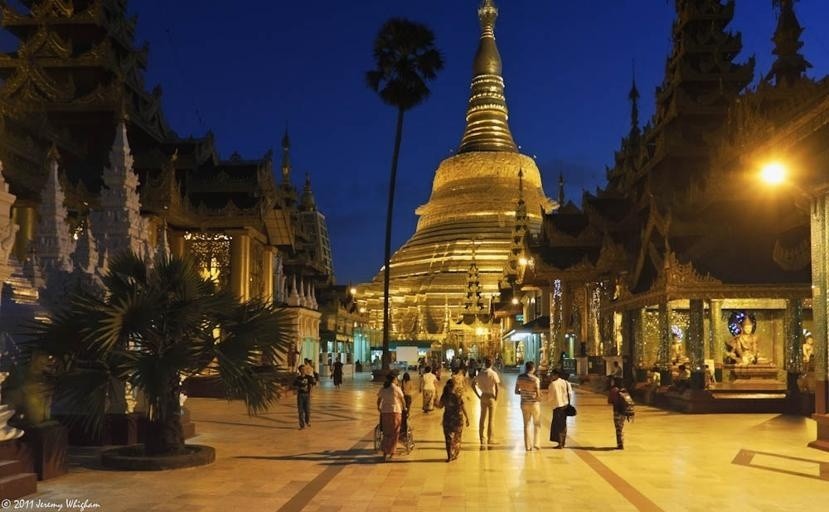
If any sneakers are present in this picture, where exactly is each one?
[615,446,625,450]
[447,454,459,463]
[298,421,313,431]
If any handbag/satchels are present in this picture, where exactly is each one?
[566,405,577,417]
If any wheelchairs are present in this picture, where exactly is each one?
[375,410,415,454]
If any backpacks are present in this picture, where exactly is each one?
[616,386,636,416]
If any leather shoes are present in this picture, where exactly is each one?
[553,444,565,448]
[480,440,498,444]
[525,444,541,450]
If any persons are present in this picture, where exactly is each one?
[292,357,715,462]
[725,311,760,366]
[802,335,814,361]
